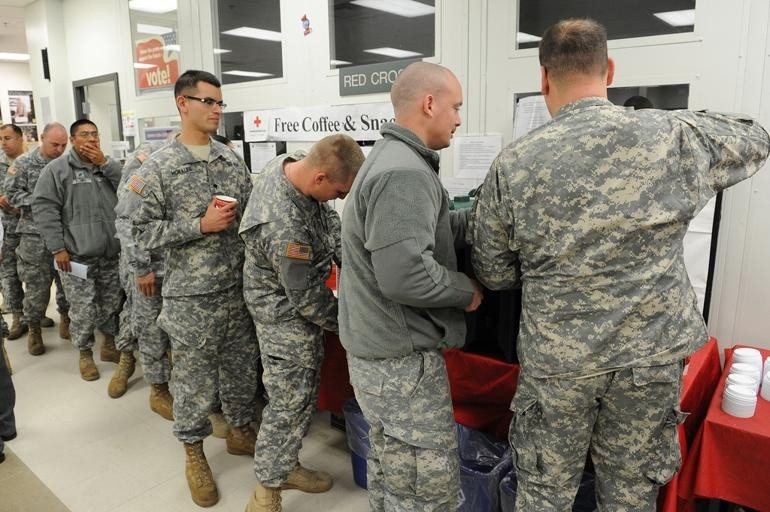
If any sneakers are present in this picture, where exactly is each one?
[1,430,17,441]
[0,452,6,464]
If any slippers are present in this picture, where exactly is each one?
[244,477,284,512]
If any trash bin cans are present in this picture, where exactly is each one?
[341,397,598,512]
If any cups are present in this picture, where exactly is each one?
[721,346,770,418]
[214,194,237,214]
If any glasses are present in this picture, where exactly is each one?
[182,94,227,111]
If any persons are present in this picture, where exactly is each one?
[114,69,261,508]
[623,96,653,110]
[337,61,484,512]
[238,134,365,512]
[466,16,770,512]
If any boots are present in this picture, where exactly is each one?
[182,439,220,508]
[59,308,95,343]
[280,460,334,494]
[26,320,46,356]
[40,315,55,328]
[225,422,258,458]
[0,301,13,315]
[107,350,136,399]
[6,311,29,341]
[78,347,100,381]
[100,340,137,364]
[148,380,176,422]
[204,409,231,439]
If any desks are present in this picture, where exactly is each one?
[674,340,770,512]
[313,318,721,512]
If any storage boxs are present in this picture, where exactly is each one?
[332,395,514,512]
[496,471,597,512]
